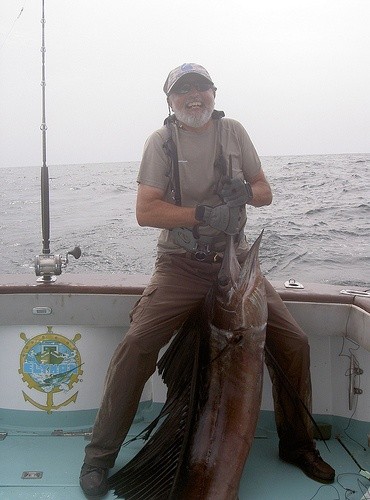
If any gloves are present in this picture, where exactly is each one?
[194,204,241,235]
[216,175,253,208]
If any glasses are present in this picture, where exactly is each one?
[170,81,212,94]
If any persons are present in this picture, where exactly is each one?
[77,62,335,500]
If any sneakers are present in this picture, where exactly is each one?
[278,442,335,484]
[78,463,109,499]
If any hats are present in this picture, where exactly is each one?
[163,62,213,94]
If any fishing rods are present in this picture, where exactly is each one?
[35,0,82,283]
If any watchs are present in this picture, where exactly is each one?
[244,180,253,204]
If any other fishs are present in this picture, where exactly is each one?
[104,155,267,500]
